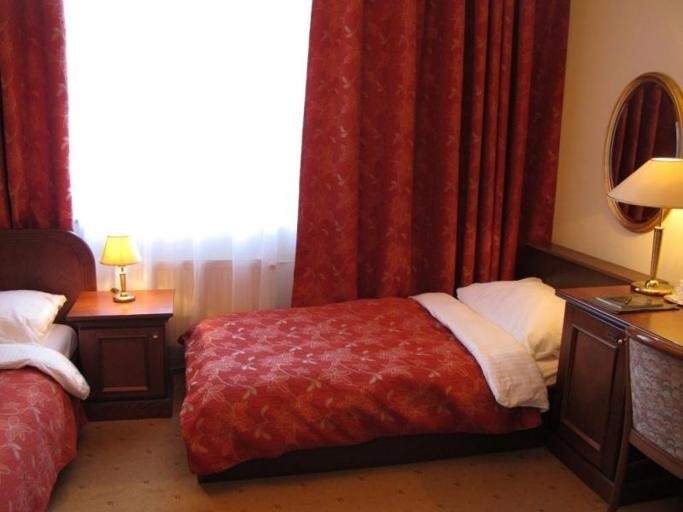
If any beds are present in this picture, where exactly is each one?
[175,274,569,488]
[0,227,100,512]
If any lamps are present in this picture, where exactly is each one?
[98,234,142,304]
[603,155,683,295]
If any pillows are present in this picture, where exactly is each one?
[1,288,67,346]
[452,275,564,360]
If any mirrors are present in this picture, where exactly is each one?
[601,72,683,238]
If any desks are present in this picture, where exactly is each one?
[546,284,682,508]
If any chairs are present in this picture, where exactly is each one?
[605,325,682,510]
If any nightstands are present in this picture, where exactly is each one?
[62,287,177,423]
